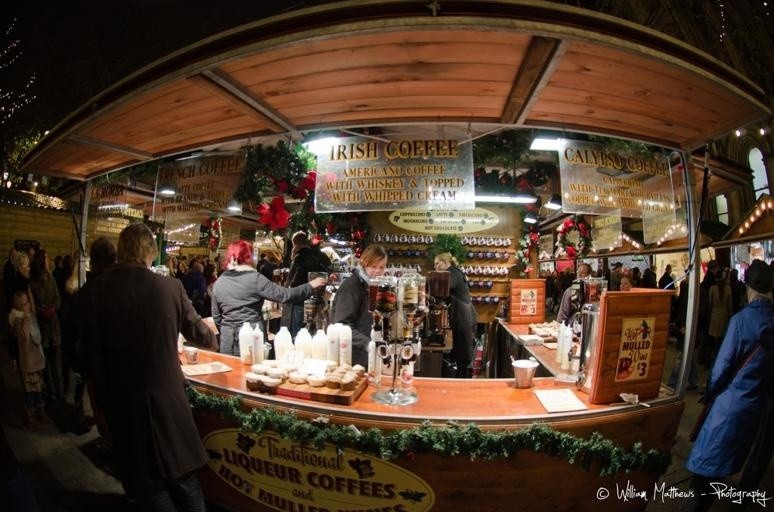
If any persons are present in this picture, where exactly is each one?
[433,251,478,378]
[330,243,388,373]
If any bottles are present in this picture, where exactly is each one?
[367,278,430,394]
[373,232,432,273]
[554,318,581,374]
[236,322,352,368]
[272,267,288,285]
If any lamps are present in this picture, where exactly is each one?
[524,211,546,226]
[543,194,562,211]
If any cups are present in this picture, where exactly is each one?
[184,348,198,363]
[460,233,510,305]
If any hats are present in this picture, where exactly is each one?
[744,259,773,294]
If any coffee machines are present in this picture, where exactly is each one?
[301,272,328,337]
[570,277,607,341]
[427,270,450,347]
